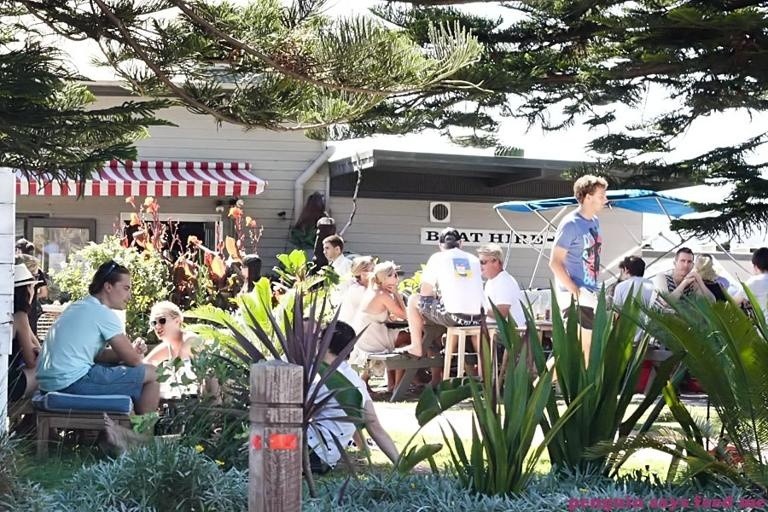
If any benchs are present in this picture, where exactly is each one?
[369,349,503,401]
[37,304,126,348]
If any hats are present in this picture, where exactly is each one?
[439,227,461,243]
[15,253,40,274]
[14,263,44,288]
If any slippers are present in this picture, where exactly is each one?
[392,347,421,360]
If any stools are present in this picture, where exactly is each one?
[442,324,494,403]
[32,391,141,463]
[500,323,543,391]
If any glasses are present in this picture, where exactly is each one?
[150,317,176,328]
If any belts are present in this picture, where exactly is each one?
[450,314,482,326]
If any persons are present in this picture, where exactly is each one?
[103,316,400,477]
[731,248,767,336]
[477,243,526,332]
[142,300,223,409]
[236,254,272,314]
[36,261,160,415]
[607,248,730,346]
[533,175,607,389]
[355,259,440,395]
[8,236,50,400]
[322,235,353,306]
[394,230,482,359]
[336,255,395,393]
